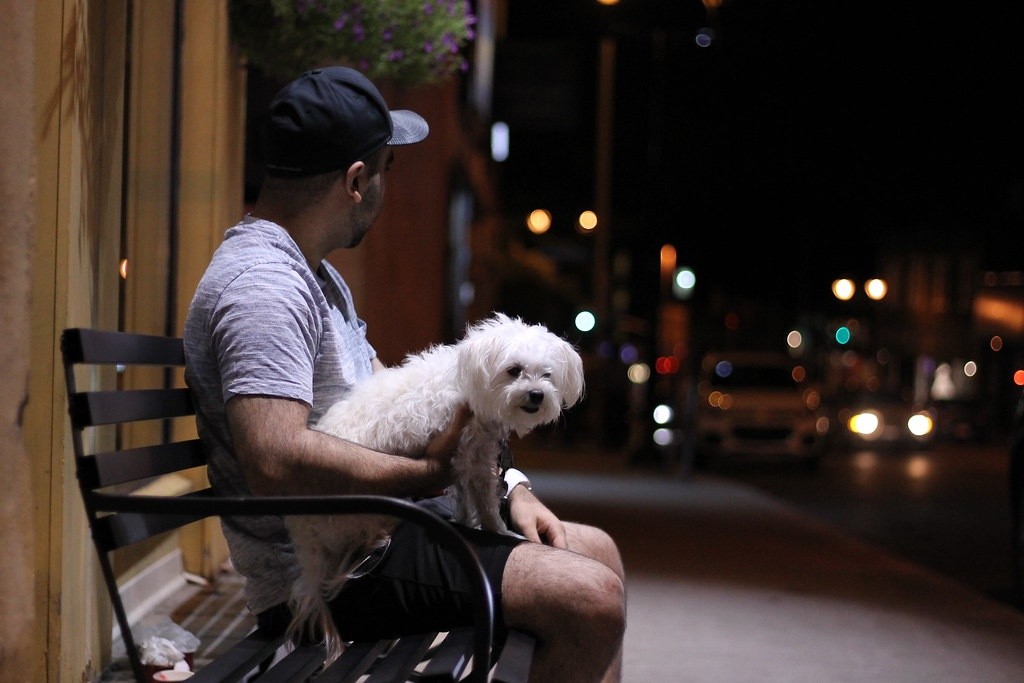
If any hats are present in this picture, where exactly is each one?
[266,65,429,170]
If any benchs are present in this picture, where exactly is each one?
[61,329,541,683]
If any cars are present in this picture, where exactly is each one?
[841,380,938,450]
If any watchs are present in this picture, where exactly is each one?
[503,468,531,501]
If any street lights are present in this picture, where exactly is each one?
[584,0,623,452]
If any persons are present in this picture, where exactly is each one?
[181,62,633,683]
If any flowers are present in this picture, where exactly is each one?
[230,0,481,92]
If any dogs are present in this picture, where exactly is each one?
[282,309,587,675]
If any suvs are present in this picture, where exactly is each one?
[688,349,830,476]
